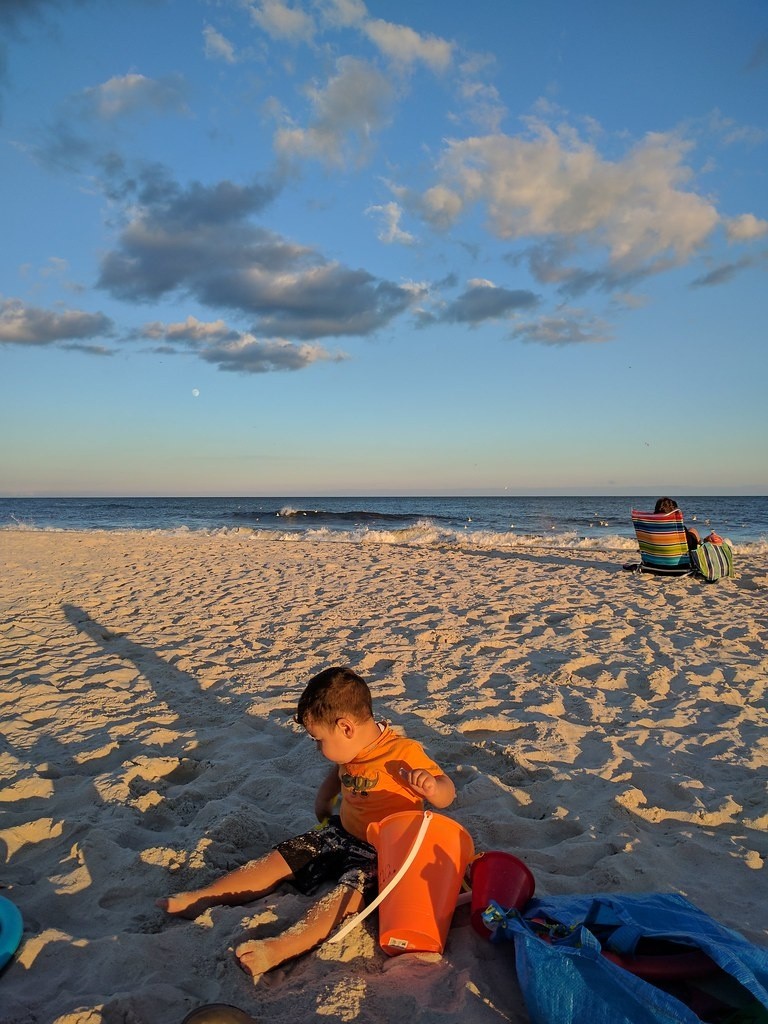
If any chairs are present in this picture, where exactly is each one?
[630,508,698,582]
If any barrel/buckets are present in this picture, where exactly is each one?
[471,853,535,936]
[368,811,474,954]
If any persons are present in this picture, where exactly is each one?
[654,497,701,555]
[153,667,456,977]
